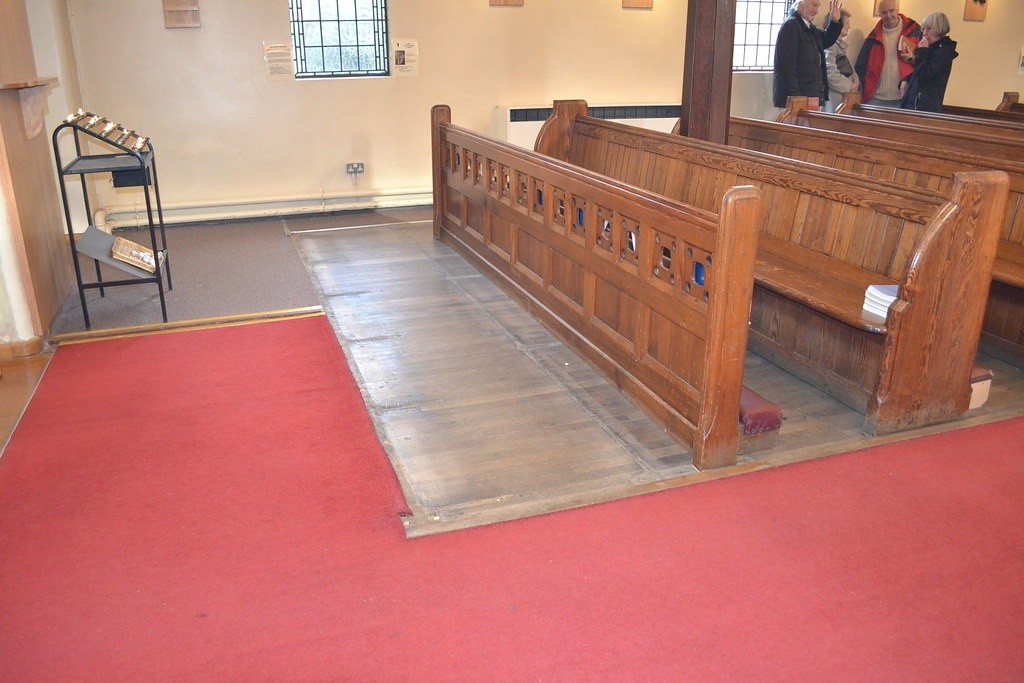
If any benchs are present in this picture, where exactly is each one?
[430,92,1024,471]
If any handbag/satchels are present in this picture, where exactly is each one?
[836,54,853,77]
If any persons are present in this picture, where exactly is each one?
[773,0,842,112]
[856,0,923,109]
[819,6,861,112]
[899,11,959,112]
